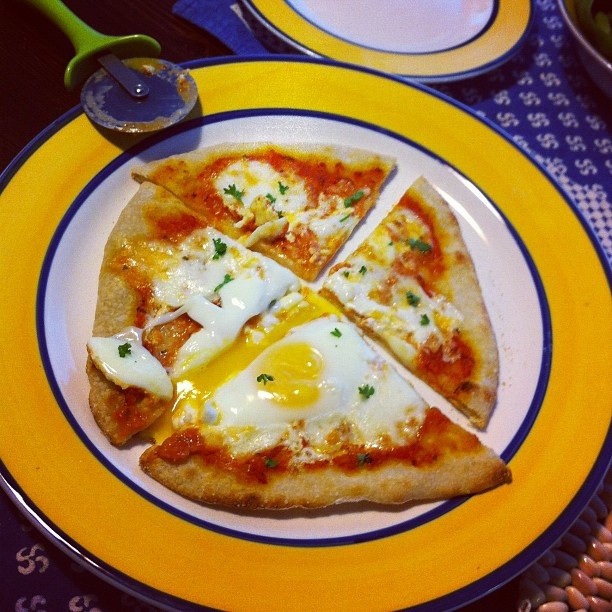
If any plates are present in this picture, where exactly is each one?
[1,51,611,612]
[241,1,537,83]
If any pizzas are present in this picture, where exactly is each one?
[85,141,513,512]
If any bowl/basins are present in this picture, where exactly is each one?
[556,1,612,98]
